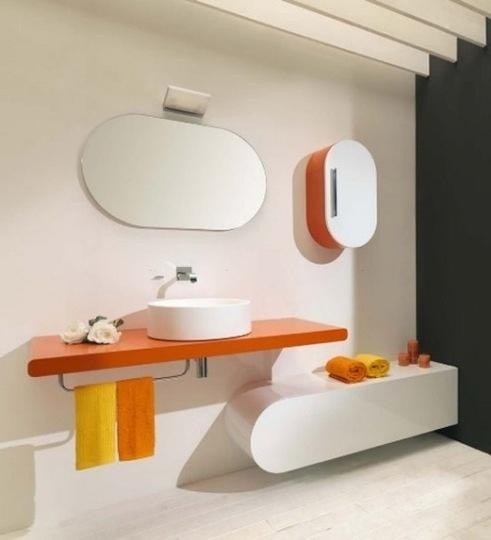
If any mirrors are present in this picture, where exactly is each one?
[80,112,268,231]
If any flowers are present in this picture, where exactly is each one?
[60,314,125,346]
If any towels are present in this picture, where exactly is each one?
[325,352,392,385]
[75,375,157,472]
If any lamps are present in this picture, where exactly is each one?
[162,84,212,118]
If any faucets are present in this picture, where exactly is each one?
[175,267,198,283]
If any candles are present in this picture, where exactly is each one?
[398,340,432,368]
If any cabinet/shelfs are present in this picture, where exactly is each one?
[322,139,379,249]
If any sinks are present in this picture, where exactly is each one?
[147,298,252,341]
